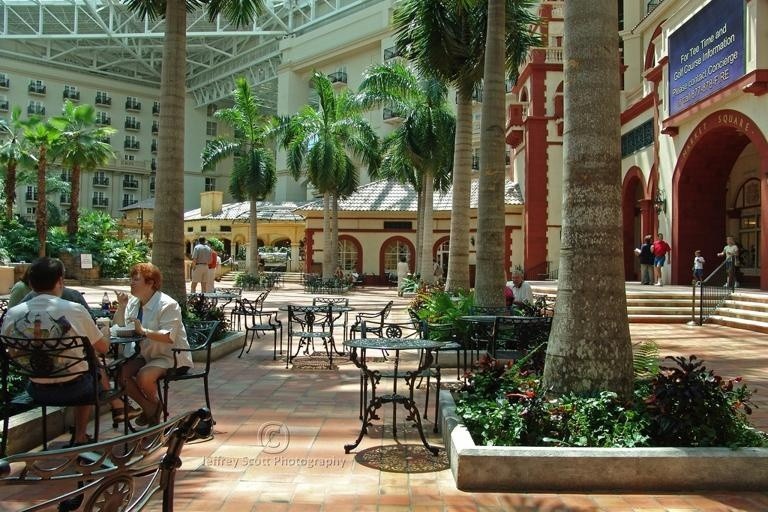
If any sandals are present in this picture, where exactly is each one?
[136,412,147,426]
[146,402,162,427]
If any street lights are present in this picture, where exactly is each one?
[137,208,143,240]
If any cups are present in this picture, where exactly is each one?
[95,320,114,337]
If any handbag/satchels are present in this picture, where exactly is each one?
[735,253,741,267]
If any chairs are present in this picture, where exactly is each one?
[360,321,441,434]
[488,316,553,358]
[0,299,10,330]
[236,298,282,361]
[212,288,244,309]
[123,320,221,437]
[235,289,272,336]
[408,308,465,390]
[231,293,265,339]
[0,334,128,459]
[349,300,394,363]
[286,304,334,369]
[532,297,547,319]
[471,306,516,364]
[0,406,215,512]
[311,297,349,355]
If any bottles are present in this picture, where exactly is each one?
[102,292,110,314]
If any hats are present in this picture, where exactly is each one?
[199,236,206,242]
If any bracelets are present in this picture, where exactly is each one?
[145,329,148,337]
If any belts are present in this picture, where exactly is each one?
[196,262,206,265]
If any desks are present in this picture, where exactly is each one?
[279,305,355,363]
[188,292,240,310]
[109,336,146,437]
[460,315,538,376]
[342,337,446,456]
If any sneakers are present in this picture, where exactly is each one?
[723,281,741,289]
[112,406,144,422]
[641,278,663,286]
[70,437,104,449]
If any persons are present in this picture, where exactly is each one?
[19,263,97,446]
[349,267,359,281]
[650,233,672,286]
[692,250,705,287]
[334,266,343,278]
[507,270,533,305]
[258,255,265,271]
[634,235,654,285]
[112,263,194,427]
[190,236,210,293]
[718,236,739,288]
[205,240,217,293]
[0,256,143,446]
[433,259,439,285]
[397,256,409,297]
[8,263,33,311]
[216,255,221,263]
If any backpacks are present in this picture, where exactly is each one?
[434,265,444,277]
